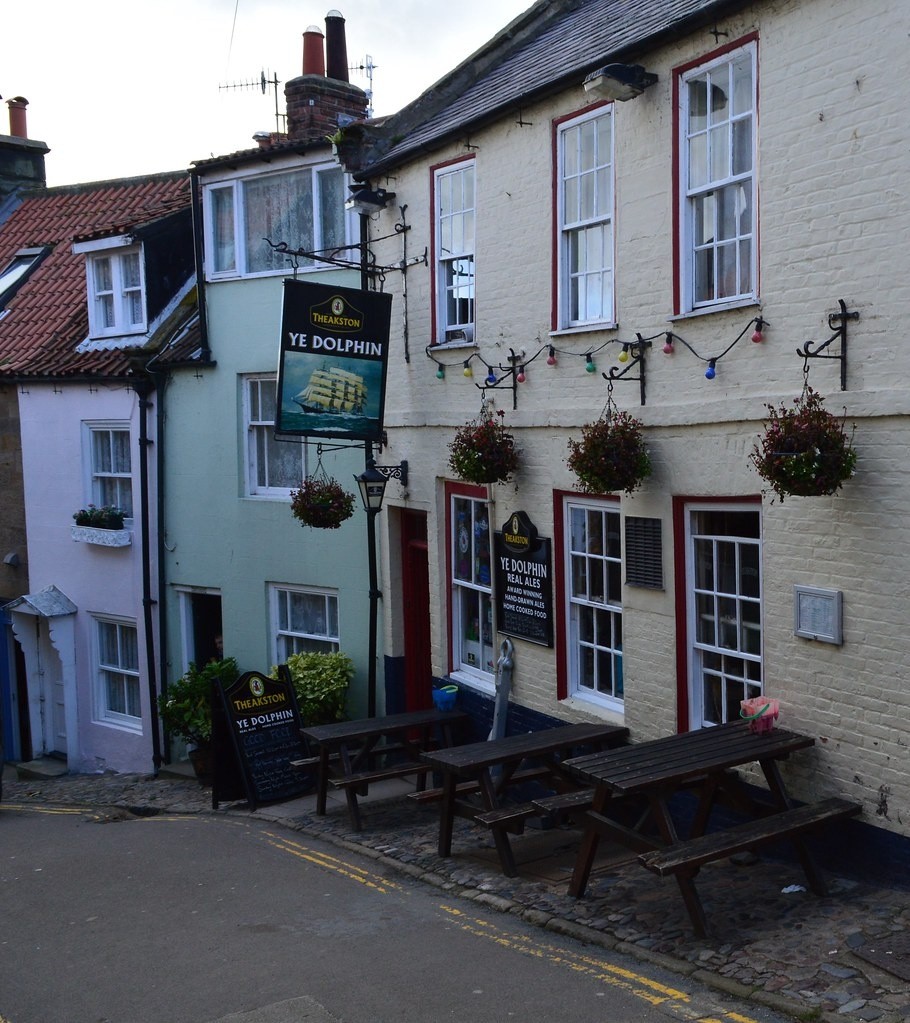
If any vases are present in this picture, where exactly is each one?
[475,476,498,484]
[76,522,123,530]
[789,482,839,498]
[188,748,218,786]
[601,484,626,492]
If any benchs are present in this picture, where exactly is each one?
[531,770,738,816]
[290,737,436,773]
[327,765,432,787]
[640,799,860,879]
[406,768,550,806]
[473,801,533,829]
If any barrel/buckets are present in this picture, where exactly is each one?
[432,685,458,712]
[739,696,779,735]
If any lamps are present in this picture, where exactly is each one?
[344,188,396,214]
[580,59,660,103]
[353,460,408,512]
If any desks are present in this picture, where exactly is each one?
[564,719,826,944]
[300,709,466,832]
[419,724,628,878]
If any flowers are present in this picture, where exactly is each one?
[746,384,859,507]
[73,501,127,522]
[445,405,521,494]
[563,408,650,500]
[288,474,356,530]
[156,652,215,747]
